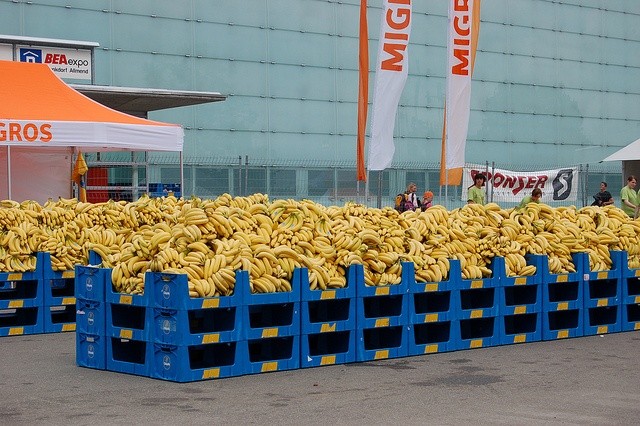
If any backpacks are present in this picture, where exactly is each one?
[418,202,431,212]
[395,192,412,211]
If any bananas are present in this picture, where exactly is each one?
[83,190,407,301]
[408,199,640,283]
[0,187,83,276]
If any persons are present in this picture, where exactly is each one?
[593,182,614,206]
[405,182,419,212]
[422,192,433,209]
[467,174,487,206]
[620,175,640,218]
[633,189,640,220]
[520,188,542,208]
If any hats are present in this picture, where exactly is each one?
[424,191,433,199]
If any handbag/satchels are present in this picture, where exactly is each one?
[417,197,422,207]
[401,201,414,211]
